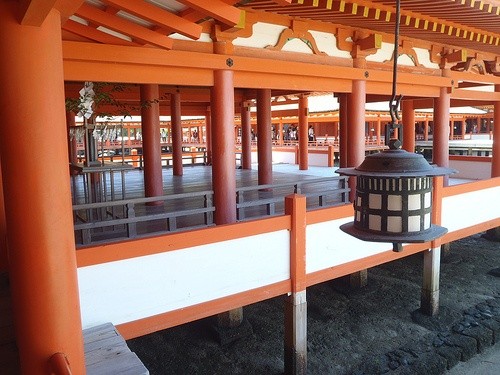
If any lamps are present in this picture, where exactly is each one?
[334,122,458,254]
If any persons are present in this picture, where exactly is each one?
[161,128,167,142]
[449,117,492,140]
[95,131,100,142]
[185,131,190,142]
[272,124,299,146]
[414,122,434,141]
[193,129,199,143]
[369,127,375,146]
[251,128,255,146]
[308,125,314,146]
[136,131,141,140]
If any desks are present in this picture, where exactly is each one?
[69,160,134,238]
